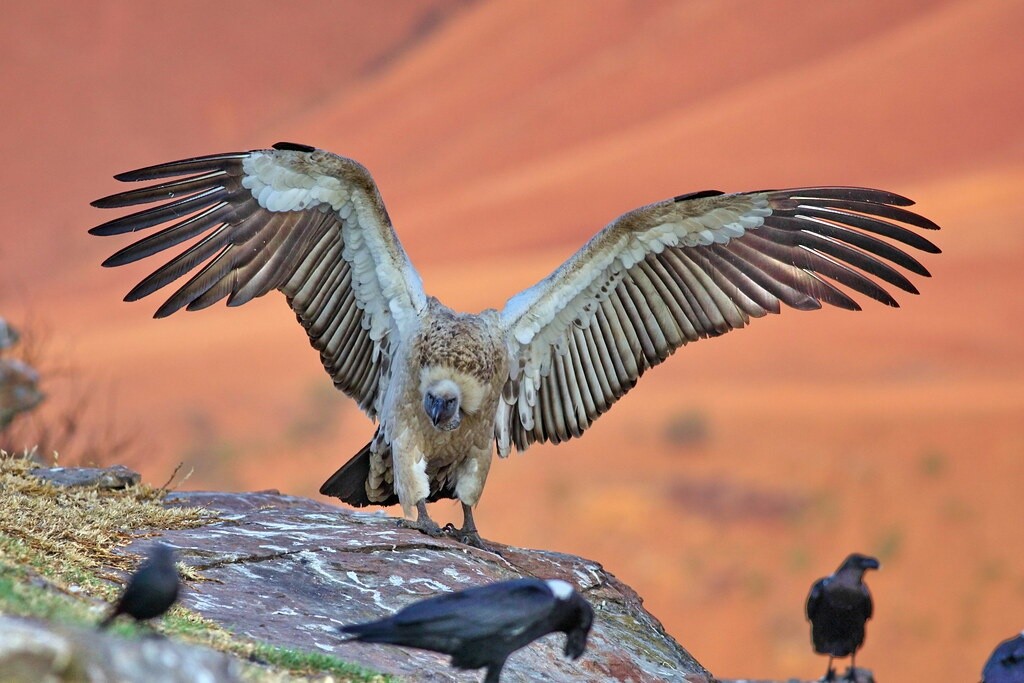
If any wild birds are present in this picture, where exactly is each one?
[95,550,182,634]
[86,139,943,549]
[804,553,880,683]
[336,577,595,683]
